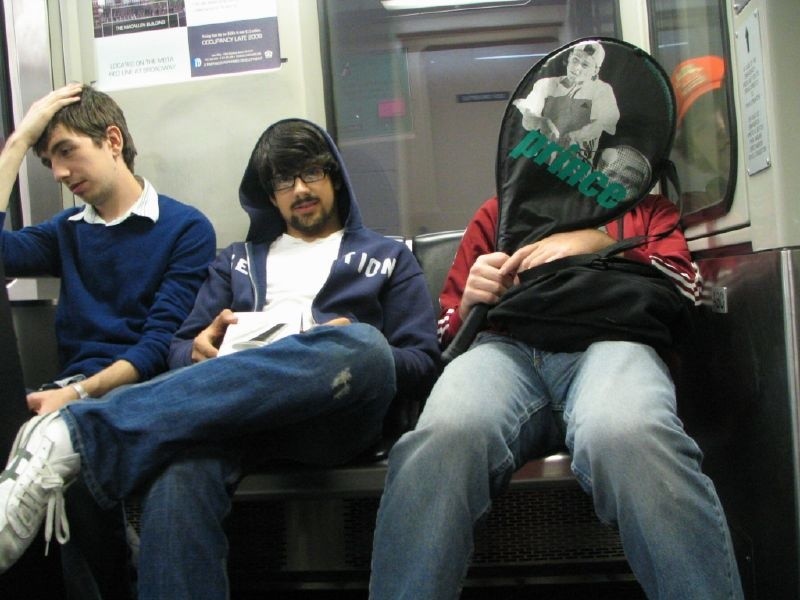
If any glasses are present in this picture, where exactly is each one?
[265,164,332,192]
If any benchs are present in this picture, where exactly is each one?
[231,230,577,500]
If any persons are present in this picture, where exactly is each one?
[512,39,620,176]
[368,192,745,600]
[0,118,443,600]
[0,81,218,474]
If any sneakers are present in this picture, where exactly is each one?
[0,410,83,571]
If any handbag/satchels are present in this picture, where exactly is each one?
[482,256,681,347]
